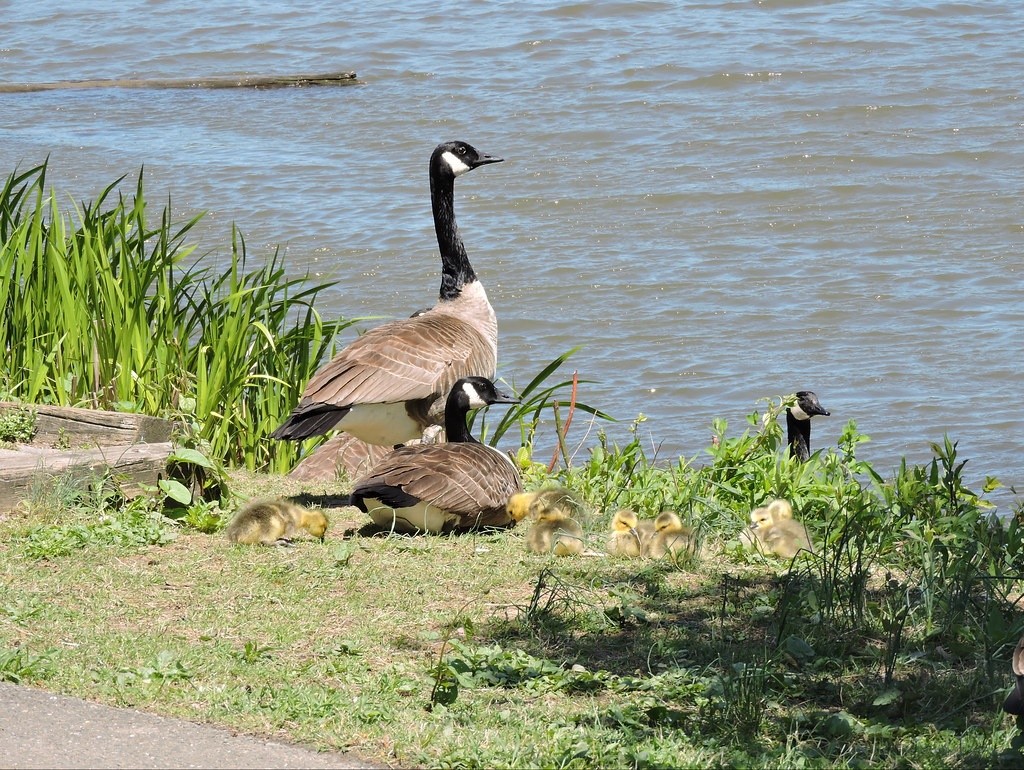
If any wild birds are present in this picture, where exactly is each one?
[265,141,506,451]
[506,485,702,563]
[225,501,329,548]
[287,376,524,536]
[739,390,831,563]
